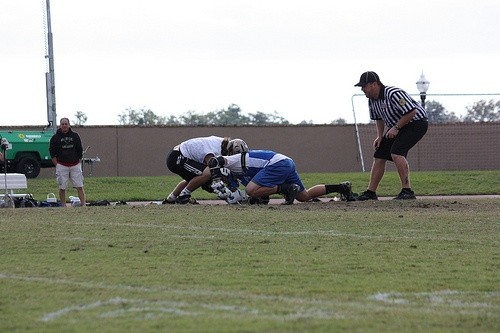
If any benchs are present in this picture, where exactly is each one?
[0,173,33,200]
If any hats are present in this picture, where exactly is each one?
[354,71,379,87]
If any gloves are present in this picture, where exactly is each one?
[227,189,250,205]
[211,181,233,199]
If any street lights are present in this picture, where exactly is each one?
[415,73,430,110]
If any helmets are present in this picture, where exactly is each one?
[232,139,249,154]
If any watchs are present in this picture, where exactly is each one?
[394,125,400,130]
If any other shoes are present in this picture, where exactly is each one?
[282,184,301,205]
[393,190,416,200]
[340,181,352,201]
[175,194,199,205]
[357,191,378,201]
[162,199,176,205]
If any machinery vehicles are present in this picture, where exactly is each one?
[0,127,58,179]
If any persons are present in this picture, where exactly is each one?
[208,149,353,205]
[354,72,429,200]
[161,135,249,206]
[49,117,86,207]
